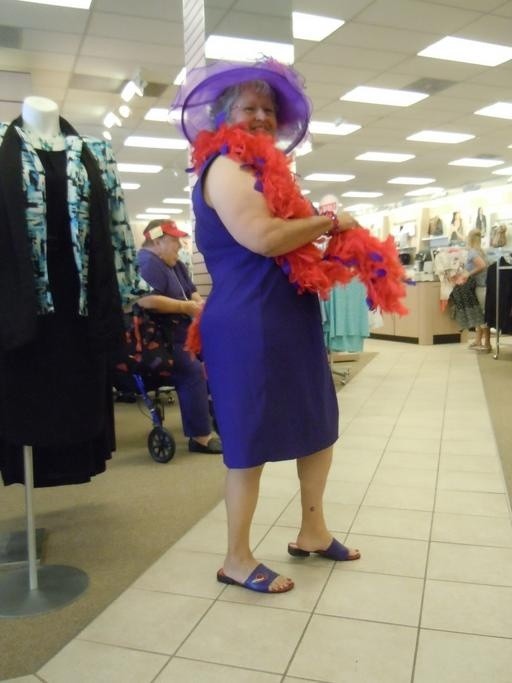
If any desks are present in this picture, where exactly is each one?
[369,281,468,345]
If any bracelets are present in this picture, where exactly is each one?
[464,272,470,279]
[321,212,338,232]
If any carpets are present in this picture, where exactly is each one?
[476,343,512,512]
[0,353,377,683]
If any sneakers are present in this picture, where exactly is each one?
[189,437,223,453]
[466,343,492,353]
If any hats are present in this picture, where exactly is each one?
[182,69,309,156]
[144,223,188,240]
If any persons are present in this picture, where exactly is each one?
[448,212,466,241]
[475,207,486,237]
[0,96,136,488]
[171,55,362,594]
[459,228,492,352]
[135,218,222,455]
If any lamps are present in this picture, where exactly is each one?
[136,83,149,98]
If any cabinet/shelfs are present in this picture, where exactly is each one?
[381,208,430,267]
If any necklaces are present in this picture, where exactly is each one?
[21,124,64,150]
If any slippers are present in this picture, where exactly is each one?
[288,536,360,561]
[217,563,293,593]
